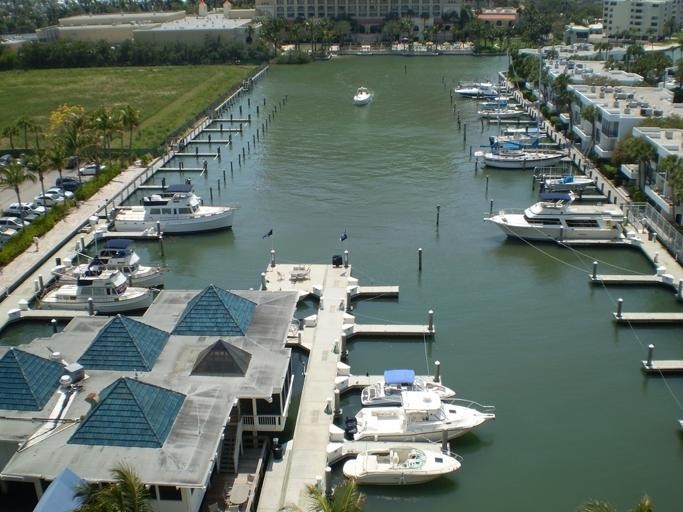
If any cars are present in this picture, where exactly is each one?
[0,153,107,246]
[541,43,586,71]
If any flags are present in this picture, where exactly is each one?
[262,229,272,238]
[339,228,348,241]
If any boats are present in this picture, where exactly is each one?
[336,377,499,487]
[351,86,371,106]
[33,181,238,316]
[450,77,630,250]
